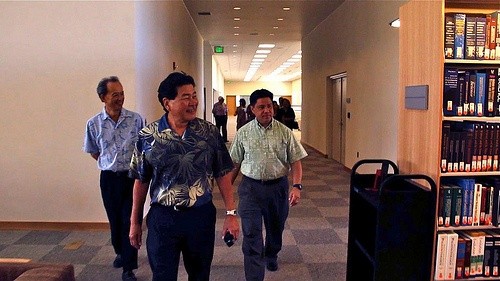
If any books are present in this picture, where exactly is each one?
[434,10,500,281]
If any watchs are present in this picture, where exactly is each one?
[226,210,237,216]
[293,184,302,190]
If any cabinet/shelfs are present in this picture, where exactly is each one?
[346,160,437,281]
[397,0,500,281]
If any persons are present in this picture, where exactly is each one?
[229,89,308,281]
[83,76,147,281]
[212,96,229,143]
[234,97,295,131]
[130,73,240,281]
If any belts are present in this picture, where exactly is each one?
[174,206,187,211]
[244,175,287,185]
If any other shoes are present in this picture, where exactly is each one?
[267,263,278,271]
[114,258,123,267]
[122,272,137,281]
